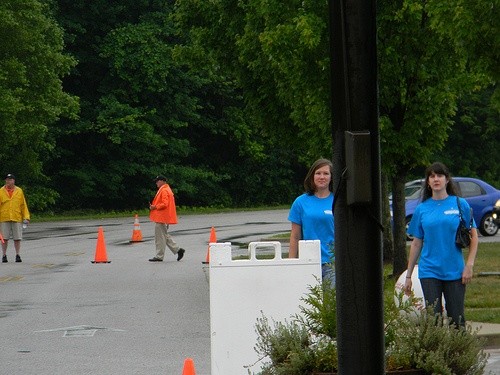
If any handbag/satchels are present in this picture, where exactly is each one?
[455,196,471,248]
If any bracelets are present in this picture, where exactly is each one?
[406,277,411,278]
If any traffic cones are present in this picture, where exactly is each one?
[91,227,111,263]
[202,227,218,264]
[129,212,145,242]
[182,358,197,375]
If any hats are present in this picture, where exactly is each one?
[5,173,15,179]
[153,176,166,182]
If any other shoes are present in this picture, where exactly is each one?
[2,255,8,263]
[149,257,163,261]
[15,255,22,262]
[177,248,185,261]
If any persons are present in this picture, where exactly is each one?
[404,162,478,335]
[0,174,30,263]
[149,175,185,261]
[287,159,336,294]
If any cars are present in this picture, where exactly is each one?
[388,177,500,241]
